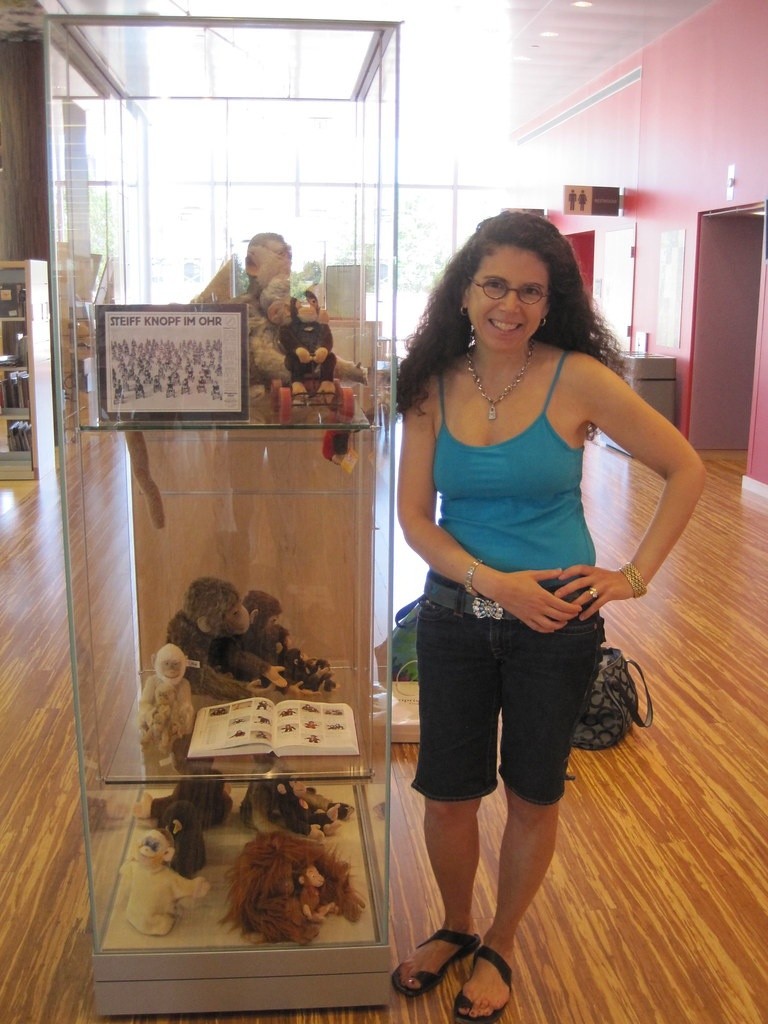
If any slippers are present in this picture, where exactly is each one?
[453,948,513,1024]
[392,928,482,997]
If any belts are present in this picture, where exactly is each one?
[425,575,592,621]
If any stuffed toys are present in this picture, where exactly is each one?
[139,576,340,743]
[218,750,366,944]
[118,734,232,945]
[120,291,367,530]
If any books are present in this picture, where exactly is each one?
[0,371,30,409]
[8,421,31,451]
[0,334,28,367]
[187,697,360,758]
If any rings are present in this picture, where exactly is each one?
[588,587,598,599]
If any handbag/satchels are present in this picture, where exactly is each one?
[570,646,653,750]
[390,595,422,681]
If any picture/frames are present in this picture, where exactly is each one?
[95,304,250,423]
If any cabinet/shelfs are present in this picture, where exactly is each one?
[44,13,401,1016]
[0,259,57,480]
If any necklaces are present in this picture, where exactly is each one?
[466,339,533,420]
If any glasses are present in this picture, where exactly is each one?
[464,275,550,304]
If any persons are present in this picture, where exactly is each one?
[393,209,707,1024]
[222,235,292,317]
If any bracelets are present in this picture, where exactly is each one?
[465,559,482,597]
[619,562,647,599]
